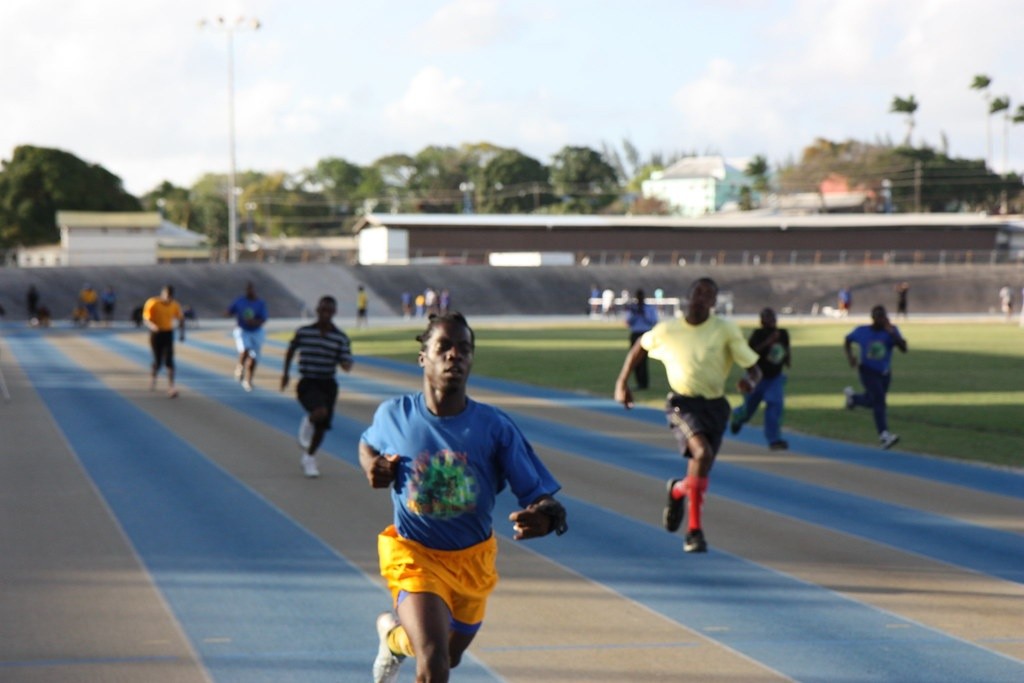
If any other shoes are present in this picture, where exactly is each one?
[235,367,244,384]
[164,388,179,401]
[149,378,157,393]
[244,382,255,393]
[770,440,788,451]
[731,414,742,435]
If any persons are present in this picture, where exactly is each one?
[141,283,186,399]
[279,296,352,478]
[842,305,908,450]
[401,286,451,320]
[627,288,658,390]
[613,278,762,552]
[728,308,794,450]
[24,283,41,327]
[78,283,101,324]
[895,282,909,320]
[356,285,369,327]
[838,282,851,319]
[100,284,116,323]
[998,285,1014,318]
[358,310,566,683]
[591,284,600,298]
[223,281,268,391]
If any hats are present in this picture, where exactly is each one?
[870,306,887,317]
[762,308,776,324]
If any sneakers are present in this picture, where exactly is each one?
[880,434,900,449]
[683,529,706,552]
[843,386,856,410]
[662,477,685,532]
[300,453,318,477]
[372,611,403,683]
[297,414,314,449]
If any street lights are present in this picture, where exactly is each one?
[199,13,263,265]
